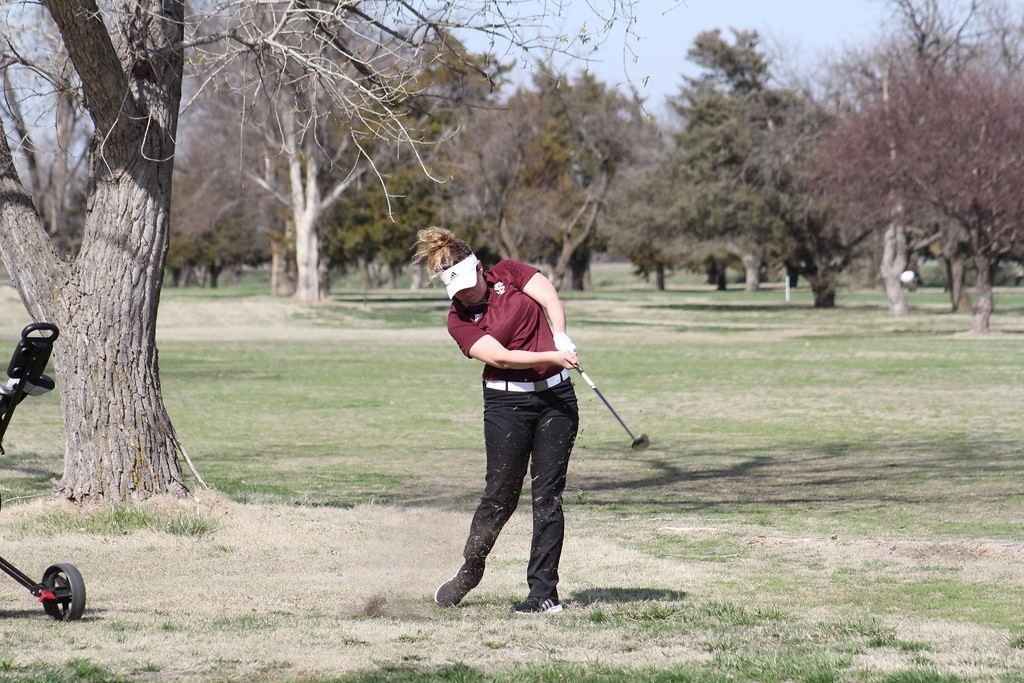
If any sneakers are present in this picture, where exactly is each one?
[436,561,470,607]
[516,594,563,615]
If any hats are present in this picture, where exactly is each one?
[440,253,479,298]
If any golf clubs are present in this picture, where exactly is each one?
[574,363,650,451]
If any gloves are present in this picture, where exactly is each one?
[553,333,576,357]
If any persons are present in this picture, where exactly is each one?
[414,227,581,620]
